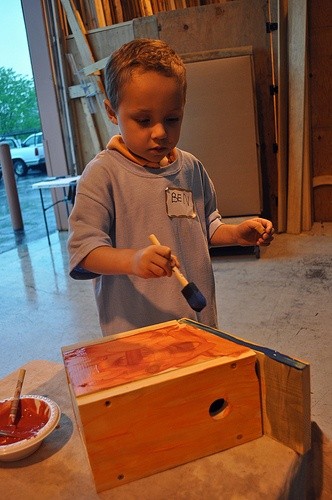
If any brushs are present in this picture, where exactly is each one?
[148,233,208,313]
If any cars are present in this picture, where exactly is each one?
[22,132,42,145]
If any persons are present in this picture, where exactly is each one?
[67,39,275,338]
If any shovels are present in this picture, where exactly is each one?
[0,367,31,439]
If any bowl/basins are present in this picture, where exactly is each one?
[0,394,62,461]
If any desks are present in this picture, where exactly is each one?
[32,176,80,246]
[0,363,323,500]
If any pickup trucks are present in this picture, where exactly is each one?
[0,136,45,177]
